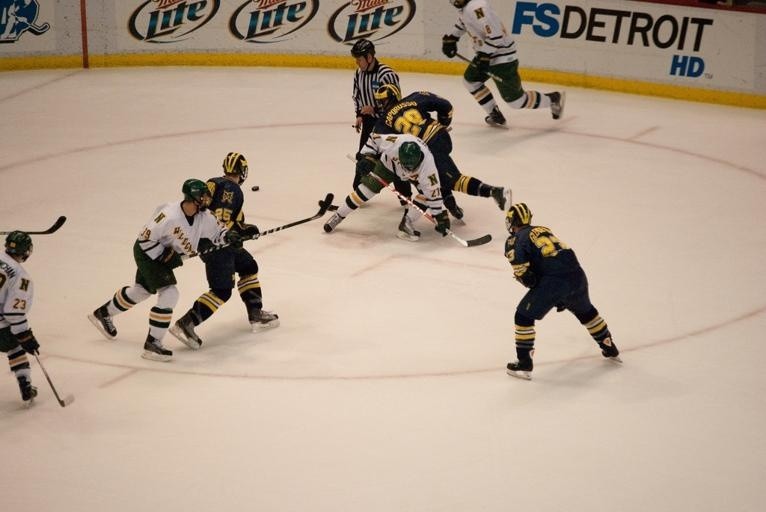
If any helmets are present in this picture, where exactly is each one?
[3,230,34,263]
[222,151,250,185]
[398,142,426,177]
[350,38,375,58]
[372,83,403,116]
[181,178,213,212]
[505,202,533,234]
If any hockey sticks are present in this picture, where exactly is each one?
[33,350,76,408]
[0,216,66,235]
[346,153,492,247]
[180,194,334,259]
[453,51,522,92]
[319,200,340,212]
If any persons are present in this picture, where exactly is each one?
[441,1,562,128]
[502,201,620,371]
[370,83,505,219]
[347,39,412,206]
[0,230,40,401]
[174,152,279,345]
[93,179,240,356]
[324,131,449,238]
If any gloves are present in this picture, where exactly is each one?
[430,209,450,238]
[442,34,458,58]
[478,51,491,73]
[163,248,183,270]
[15,328,41,356]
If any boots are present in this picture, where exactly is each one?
[595,332,620,359]
[247,308,280,325]
[484,104,506,127]
[323,211,346,234]
[491,185,506,211]
[398,208,422,237]
[92,299,118,337]
[16,375,38,401]
[544,91,562,120]
[506,347,535,372]
[143,327,174,356]
[175,309,205,346]
